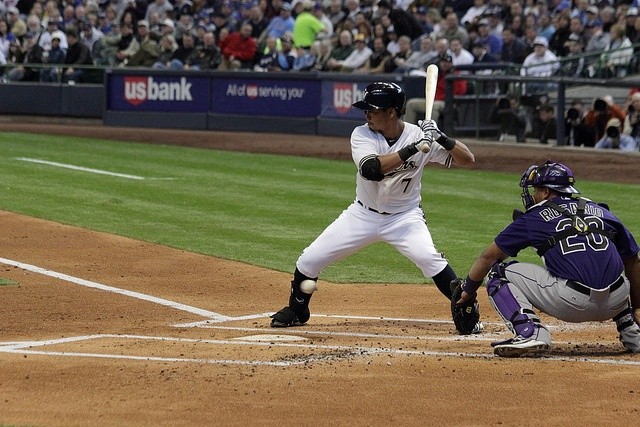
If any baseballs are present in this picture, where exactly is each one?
[302,279,317,294]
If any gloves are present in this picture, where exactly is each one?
[417,119,444,138]
[416,136,433,149]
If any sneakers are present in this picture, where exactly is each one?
[620,334,639,354]
[493,336,548,354]
[473,321,486,333]
[271,304,309,325]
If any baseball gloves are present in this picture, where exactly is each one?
[449,280,480,334]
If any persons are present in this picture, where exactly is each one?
[450,160,639,356]
[0,1,639,88]
[268,81,484,334]
[489,89,640,152]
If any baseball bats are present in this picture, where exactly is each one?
[423,64,438,153]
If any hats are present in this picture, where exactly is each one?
[46,17,62,25]
[352,81,407,111]
[352,33,365,42]
[587,5,599,17]
[627,6,638,17]
[8,7,20,14]
[519,160,580,195]
[281,2,292,10]
[534,36,548,47]
[606,119,621,126]
[160,18,174,29]
[479,19,488,25]
[136,20,148,25]
